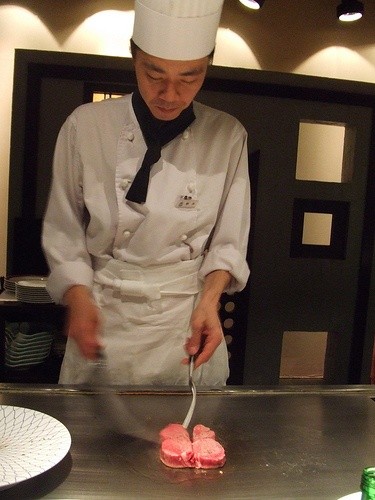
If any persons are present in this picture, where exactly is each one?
[41,0,252,387]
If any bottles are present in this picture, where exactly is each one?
[360,466,375,500]
[48,328,67,363]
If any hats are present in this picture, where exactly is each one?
[133,0,224,60]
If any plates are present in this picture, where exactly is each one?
[4,275,48,293]
[15,280,52,304]
[0,404,72,489]
[4,322,56,368]
